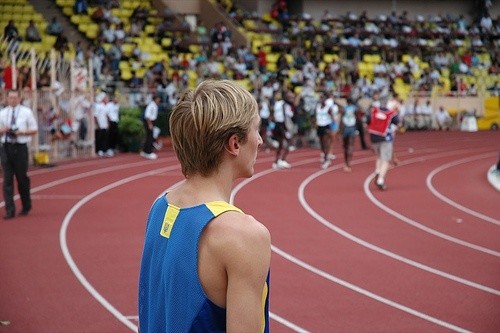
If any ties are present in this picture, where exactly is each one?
[11,105,15,126]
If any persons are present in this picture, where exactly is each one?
[138,80,271,333]
[368,96,402,190]
[0,90,38,219]
[0,0,500,169]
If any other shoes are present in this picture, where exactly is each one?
[332,154,336,159]
[98,150,104,157]
[106,149,114,157]
[140,149,156,160]
[288,145,296,151]
[320,152,325,162]
[374,174,387,190]
[276,159,292,169]
[321,159,332,168]
